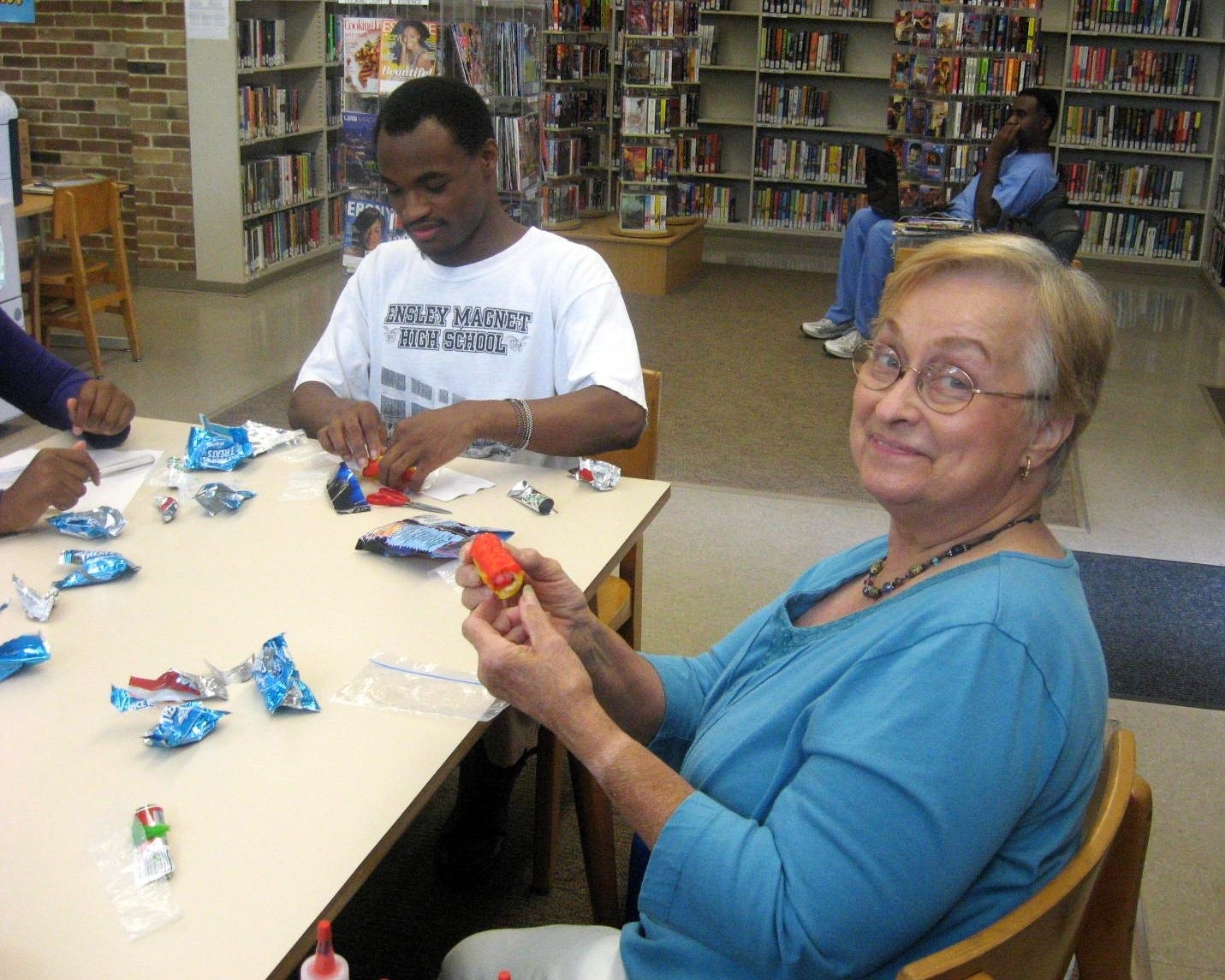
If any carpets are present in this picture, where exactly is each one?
[1072,550,1225,712]
[1195,381,1225,440]
[193,257,1093,534]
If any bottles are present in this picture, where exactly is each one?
[300,919,350,980]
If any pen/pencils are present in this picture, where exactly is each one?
[85,454,155,482]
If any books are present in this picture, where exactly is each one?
[240,150,320,274]
[342,18,438,270]
[236,16,300,142]
[448,0,871,232]
[885,0,1225,289]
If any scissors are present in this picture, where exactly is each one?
[366,488,452,514]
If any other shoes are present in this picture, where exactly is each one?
[435,753,524,888]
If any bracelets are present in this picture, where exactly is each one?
[501,397,533,455]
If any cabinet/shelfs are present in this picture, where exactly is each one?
[184,1,1225,316]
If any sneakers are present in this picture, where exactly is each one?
[822,330,870,359]
[800,318,856,340]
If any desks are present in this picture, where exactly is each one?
[0,416,671,980]
[15,177,135,351]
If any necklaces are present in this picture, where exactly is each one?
[863,512,1042,600]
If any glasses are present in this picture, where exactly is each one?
[851,342,1055,415]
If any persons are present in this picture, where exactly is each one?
[895,12,913,45]
[899,188,912,207]
[0,306,135,538]
[917,14,932,47]
[288,78,647,884]
[391,20,434,79]
[937,113,946,137]
[344,207,386,257]
[436,233,1114,980]
[934,113,942,138]
[912,12,930,46]
[906,143,922,174]
[799,87,1060,360]
[910,184,924,207]
[934,61,951,95]
[892,97,907,133]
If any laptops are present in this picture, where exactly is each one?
[864,146,947,223]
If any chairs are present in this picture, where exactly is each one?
[896,723,1152,980]
[532,368,659,895]
[17,177,141,381]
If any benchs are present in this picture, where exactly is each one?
[893,180,1083,277]
